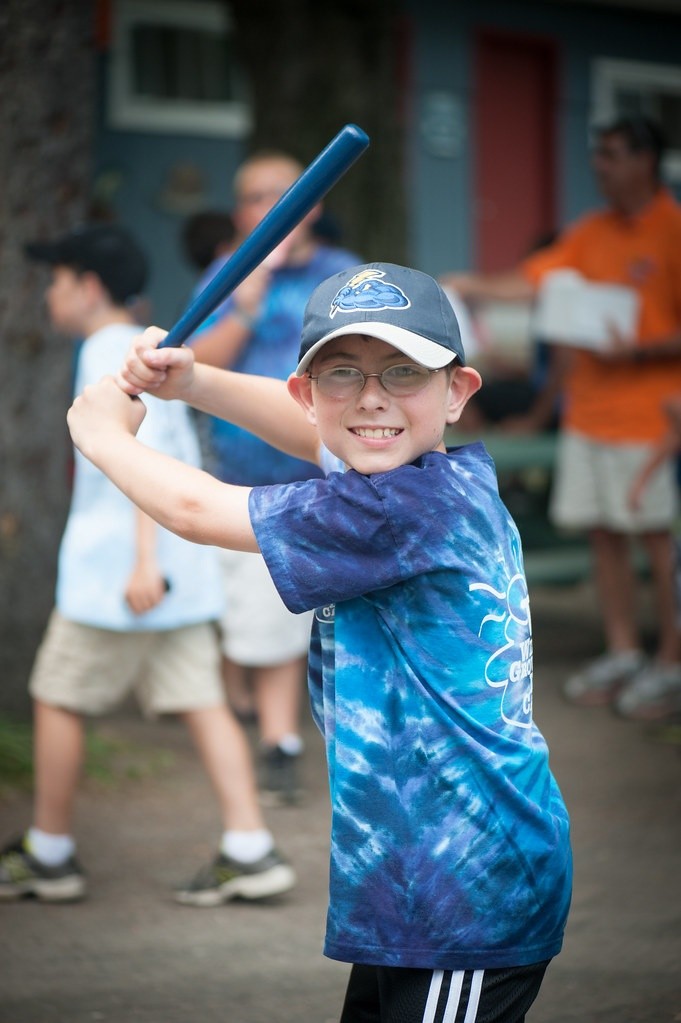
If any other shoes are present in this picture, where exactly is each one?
[564,650,644,707]
[254,739,310,805]
[615,659,681,721]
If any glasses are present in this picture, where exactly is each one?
[306,362,454,400]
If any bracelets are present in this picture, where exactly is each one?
[218,301,255,334]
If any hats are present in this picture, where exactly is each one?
[295,261,465,378]
[22,223,146,301]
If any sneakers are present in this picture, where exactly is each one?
[0,830,87,903]
[169,846,296,907]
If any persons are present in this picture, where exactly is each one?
[178,206,281,730]
[0,213,303,906]
[168,206,604,713]
[438,114,679,722]
[62,261,573,1023]
[178,149,366,775]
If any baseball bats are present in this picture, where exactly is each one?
[127,121,373,405]
